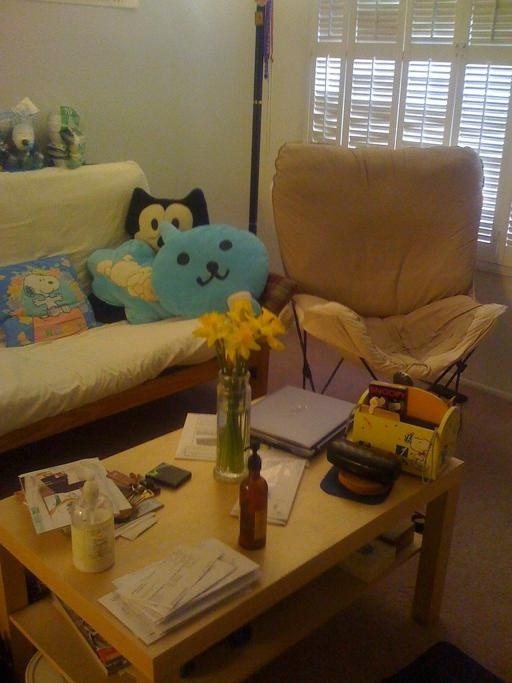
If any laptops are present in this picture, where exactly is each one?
[247,383,356,457]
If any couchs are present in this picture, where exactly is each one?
[0,156,274,463]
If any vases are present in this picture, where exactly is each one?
[212,362,256,481]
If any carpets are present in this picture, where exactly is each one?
[390,639,505,683]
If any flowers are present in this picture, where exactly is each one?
[190,287,287,472]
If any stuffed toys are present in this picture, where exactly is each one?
[1,118,46,171]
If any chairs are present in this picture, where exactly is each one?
[269,141,503,406]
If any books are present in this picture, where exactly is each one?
[18,455,165,541]
[51,591,131,676]
[230,445,308,526]
[368,378,408,420]
[360,403,399,423]
